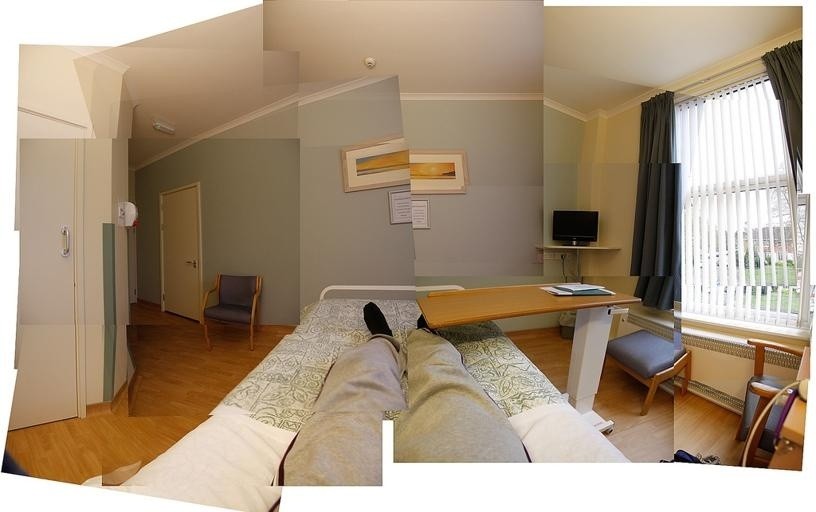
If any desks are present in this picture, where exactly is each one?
[544,244,621,284]
[414,282,642,439]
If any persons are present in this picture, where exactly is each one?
[267,301,532,511]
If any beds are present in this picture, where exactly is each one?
[155,282,632,465]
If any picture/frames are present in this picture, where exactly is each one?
[408,149,469,195]
[340,136,410,193]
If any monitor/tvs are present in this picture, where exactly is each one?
[553,210,598,246]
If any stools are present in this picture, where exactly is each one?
[601,329,692,416]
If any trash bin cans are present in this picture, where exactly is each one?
[559,311,577,340]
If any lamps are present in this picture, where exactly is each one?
[152,123,175,135]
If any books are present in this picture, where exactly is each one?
[538,283,617,297]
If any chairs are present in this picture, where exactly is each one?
[735,338,803,449]
[199,275,264,350]
